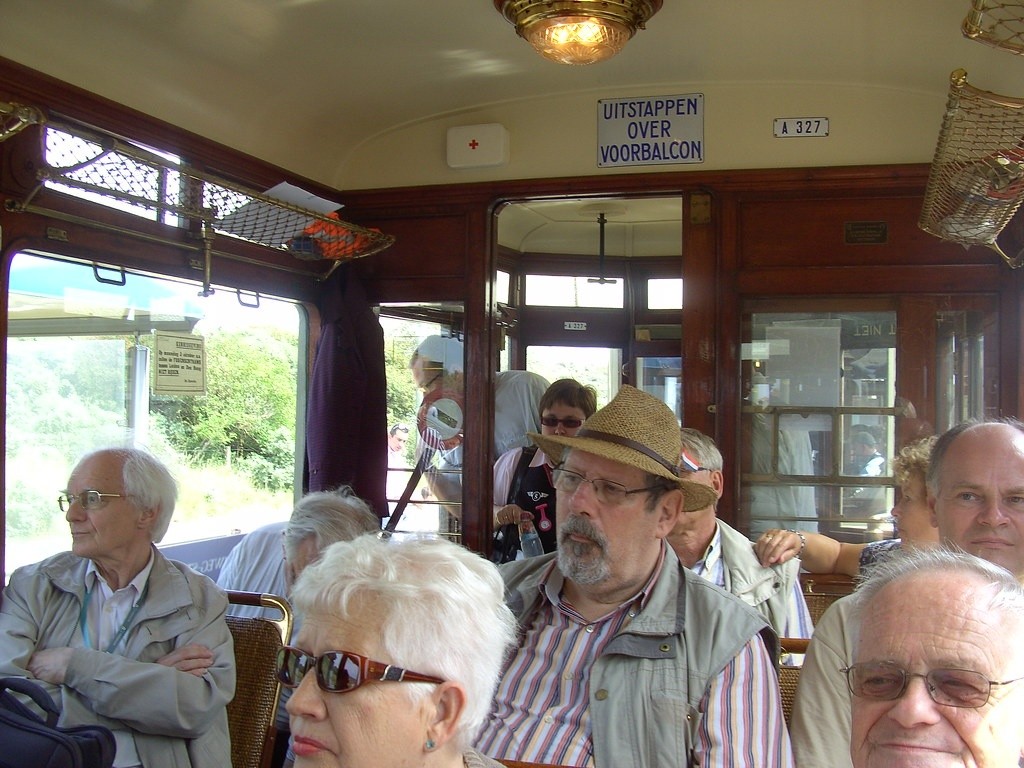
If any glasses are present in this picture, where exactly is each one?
[550,461,678,505]
[538,416,585,428]
[838,660,1021,709]
[413,372,444,394]
[57,490,133,512]
[273,646,445,696]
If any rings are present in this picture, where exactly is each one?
[501,515,507,520]
[766,534,773,539]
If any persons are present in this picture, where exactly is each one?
[386,421,435,526]
[747,373,888,545]
[275,529,521,768]
[470,383,798,768]
[216,484,381,732]
[667,427,815,667]
[753,416,1023,768]
[407,334,597,564]
[0,447,237,768]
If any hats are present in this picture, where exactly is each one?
[525,385,717,512]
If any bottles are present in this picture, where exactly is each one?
[517,513,545,559]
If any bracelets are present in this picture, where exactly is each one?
[783,529,805,559]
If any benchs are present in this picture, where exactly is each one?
[224,590,292,768]
[776,572,862,722]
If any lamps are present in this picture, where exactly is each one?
[493,0,664,65]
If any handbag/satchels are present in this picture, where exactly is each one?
[0,676,117,768]
